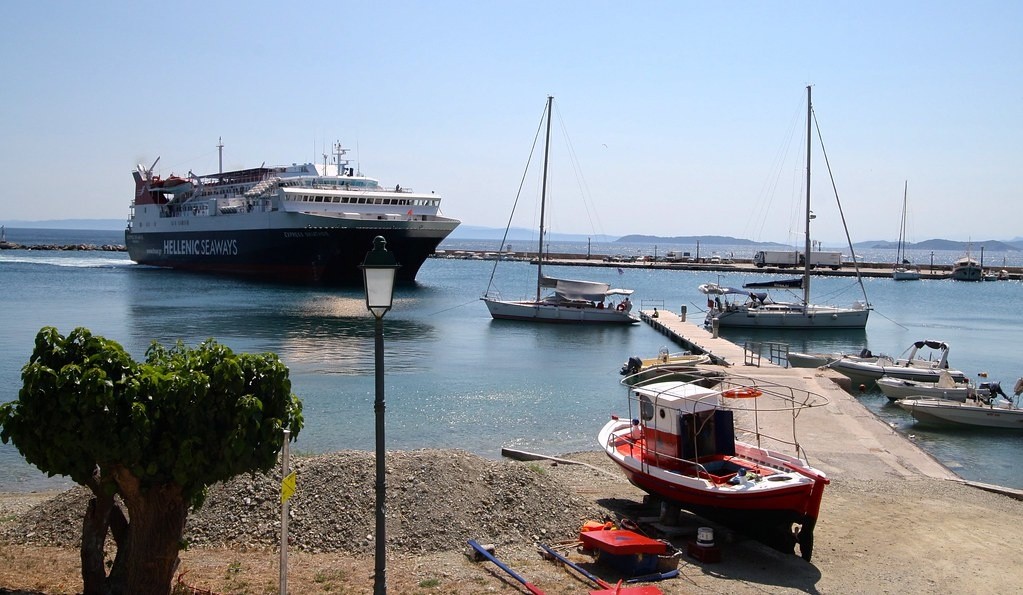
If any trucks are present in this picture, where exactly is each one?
[665,251,682,262]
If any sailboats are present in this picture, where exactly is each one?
[893,179,922,281]
[480,93,643,324]
[704,84,871,328]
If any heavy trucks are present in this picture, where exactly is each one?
[754,249,841,271]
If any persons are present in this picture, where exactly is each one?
[621,297,631,307]
[597,301,604,309]
[652,308,659,318]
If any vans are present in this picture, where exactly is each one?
[711,256,720,263]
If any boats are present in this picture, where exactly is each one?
[893,378,1023,430]
[597,363,831,563]
[620,345,712,372]
[163,174,186,188]
[999,256,1010,280]
[124,134,461,289]
[787,334,968,393]
[984,270,996,281]
[875,367,1014,407]
[953,237,982,281]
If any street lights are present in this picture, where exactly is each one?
[361,230,402,595]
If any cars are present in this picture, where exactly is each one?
[687,257,695,262]
[723,259,733,263]
[603,254,645,263]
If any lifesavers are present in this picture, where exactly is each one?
[721,388,763,399]
[616,304,625,311]
